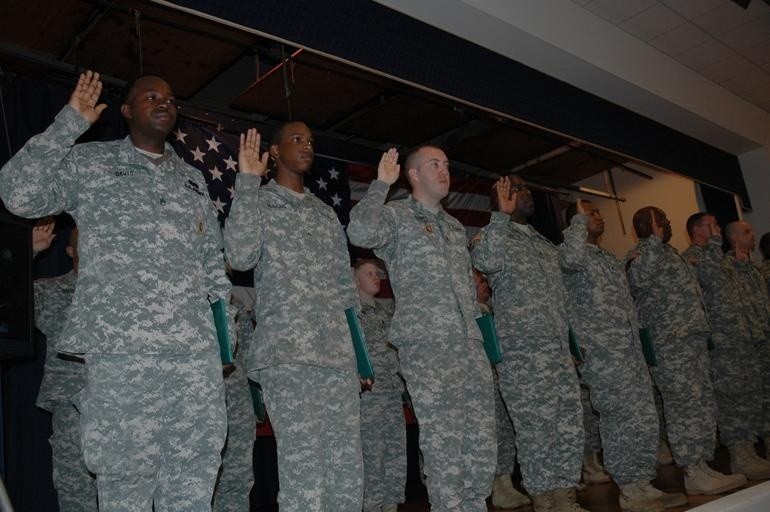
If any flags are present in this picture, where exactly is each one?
[161,117,496,300]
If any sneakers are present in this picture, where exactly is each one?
[655,447,673,465]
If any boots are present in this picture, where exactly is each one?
[619,481,665,512]
[553,486,592,512]
[683,464,739,497]
[640,479,688,508]
[728,441,770,480]
[696,460,747,487]
[531,492,562,512]
[583,455,612,485]
[492,475,531,511]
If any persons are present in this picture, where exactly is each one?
[346,144,498,512]
[556,198,688,510]
[759,231,769,284]
[566,349,613,483]
[682,210,770,479]
[348,258,410,511]
[470,175,588,511]
[2,70,239,511]
[626,207,748,495]
[30,216,101,509]
[644,360,676,466]
[221,120,375,512]
[723,218,770,336]
[471,269,535,509]
[213,257,259,511]
